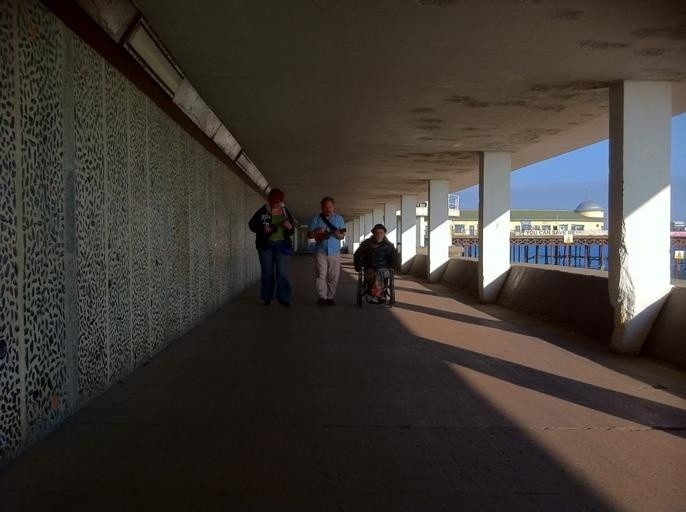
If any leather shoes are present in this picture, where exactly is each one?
[318,298,326,306]
[327,299,336,307]
[264,299,271,306]
[282,301,291,306]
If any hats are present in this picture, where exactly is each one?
[371,224,387,233]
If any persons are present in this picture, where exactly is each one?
[306,196,347,306]
[352,223,401,305]
[248,187,295,308]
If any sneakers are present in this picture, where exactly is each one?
[371,295,386,303]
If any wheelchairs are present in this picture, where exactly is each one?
[354,260,398,309]
